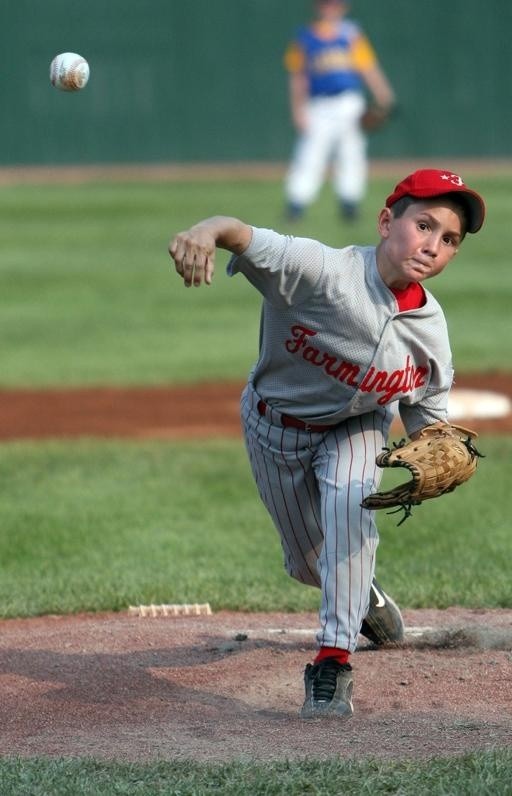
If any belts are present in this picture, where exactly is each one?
[258,401,339,432]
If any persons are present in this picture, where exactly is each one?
[284,0,396,224]
[168,168,487,723]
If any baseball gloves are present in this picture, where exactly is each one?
[360,101,391,131]
[360,421,487,525]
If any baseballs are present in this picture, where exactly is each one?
[49,51,89,90]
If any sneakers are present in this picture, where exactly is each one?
[300,656,354,721]
[360,574,405,648]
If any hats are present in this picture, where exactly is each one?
[386,169,486,234]
